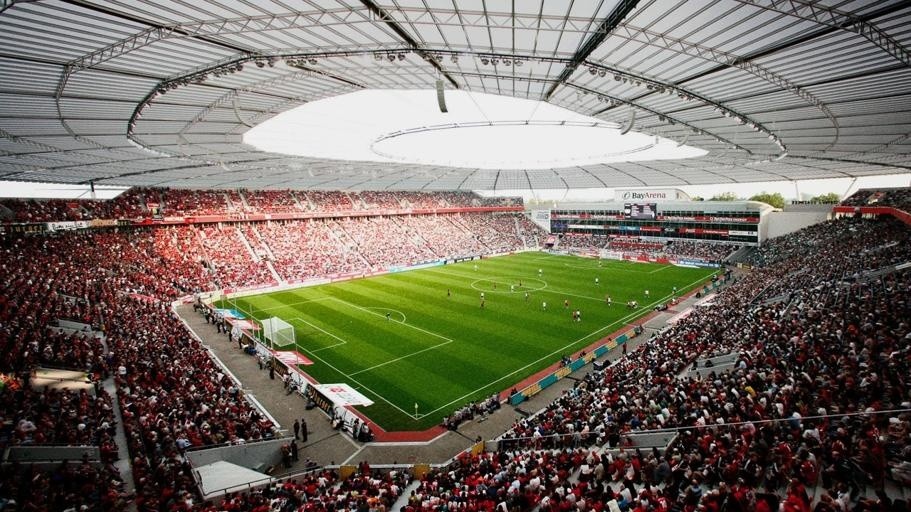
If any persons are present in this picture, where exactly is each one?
[0,187,910,511]
